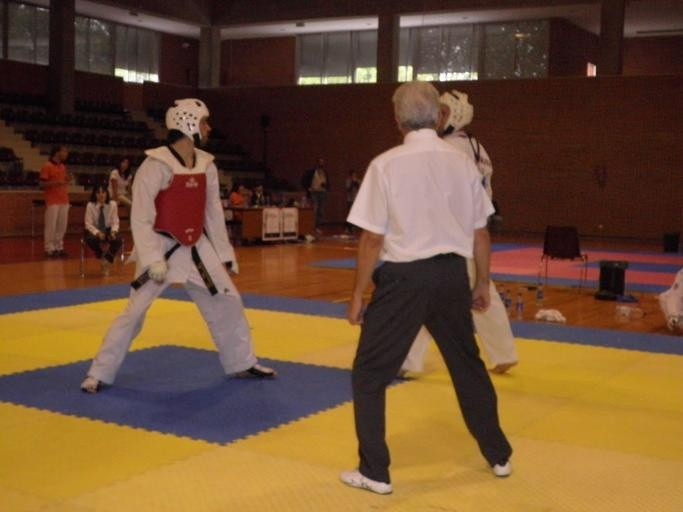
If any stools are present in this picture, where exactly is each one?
[79,234,124,279]
[118,204,129,225]
[226,218,248,247]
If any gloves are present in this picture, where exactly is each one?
[148,262,169,284]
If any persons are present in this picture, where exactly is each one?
[394,87,522,382]
[105,157,133,208]
[249,181,273,206]
[38,144,74,259]
[344,169,361,234]
[339,79,513,493]
[81,97,279,394]
[82,181,123,274]
[300,157,330,234]
[227,181,247,222]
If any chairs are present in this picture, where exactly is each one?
[1,89,280,193]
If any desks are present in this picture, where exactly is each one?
[223,206,316,246]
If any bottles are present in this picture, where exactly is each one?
[499,282,543,320]
[616,306,647,319]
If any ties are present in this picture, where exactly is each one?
[98,205,107,233]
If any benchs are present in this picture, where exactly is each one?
[31,199,89,237]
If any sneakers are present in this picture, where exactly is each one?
[230,364,278,378]
[43,250,73,258]
[80,376,104,393]
[339,470,393,495]
[492,462,511,477]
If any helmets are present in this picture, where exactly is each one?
[440,90,474,134]
[165,98,210,141]
